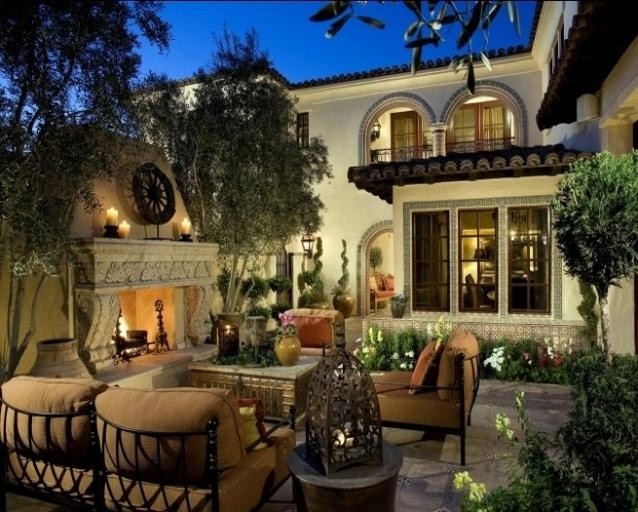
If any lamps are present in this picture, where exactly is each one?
[305,320,383,480]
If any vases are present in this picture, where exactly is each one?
[275,335,301,366]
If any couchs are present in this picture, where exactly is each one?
[0,374,296,512]
[370,272,395,313]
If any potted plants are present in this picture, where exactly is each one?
[390,295,409,318]
[332,239,355,318]
[303,237,330,310]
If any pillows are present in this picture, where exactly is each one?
[292,316,334,348]
[236,399,272,450]
[409,338,442,395]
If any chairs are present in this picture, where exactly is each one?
[375,328,485,466]
[282,309,345,357]
[465,274,493,309]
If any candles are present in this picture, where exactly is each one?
[180,217,191,234]
[117,220,131,238]
[106,206,118,226]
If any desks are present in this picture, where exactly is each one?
[487,291,495,300]
[287,438,404,512]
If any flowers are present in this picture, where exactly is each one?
[278,311,299,334]
[275,313,299,336]
